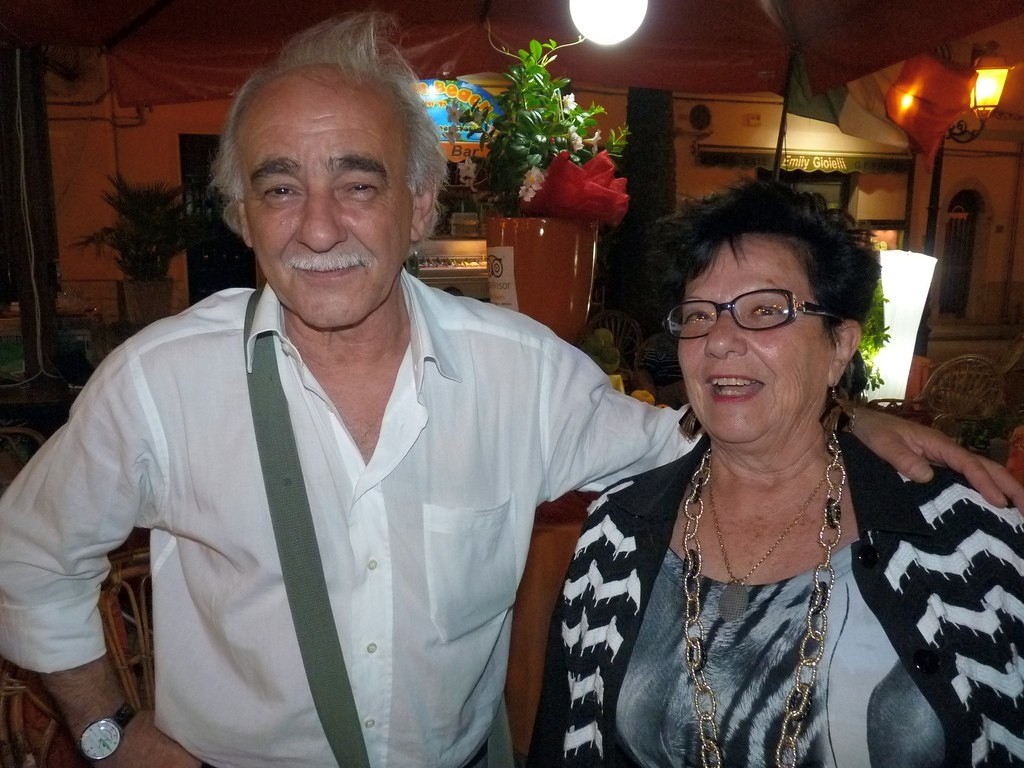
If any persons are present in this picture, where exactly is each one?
[530,175,1024,768]
[1,15,1022,768]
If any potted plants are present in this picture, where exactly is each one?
[65,169,211,329]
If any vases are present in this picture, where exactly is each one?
[482,217,598,342]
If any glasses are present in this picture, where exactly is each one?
[662,288,843,340]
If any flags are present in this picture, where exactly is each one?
[789,25,979,171]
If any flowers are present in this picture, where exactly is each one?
[445,36,634,228]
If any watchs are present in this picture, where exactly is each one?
[75,702,135,768]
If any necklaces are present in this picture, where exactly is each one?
[708,460,833,620]
[685,432,846,768]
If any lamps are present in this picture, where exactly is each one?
[885,38,1019,356]
